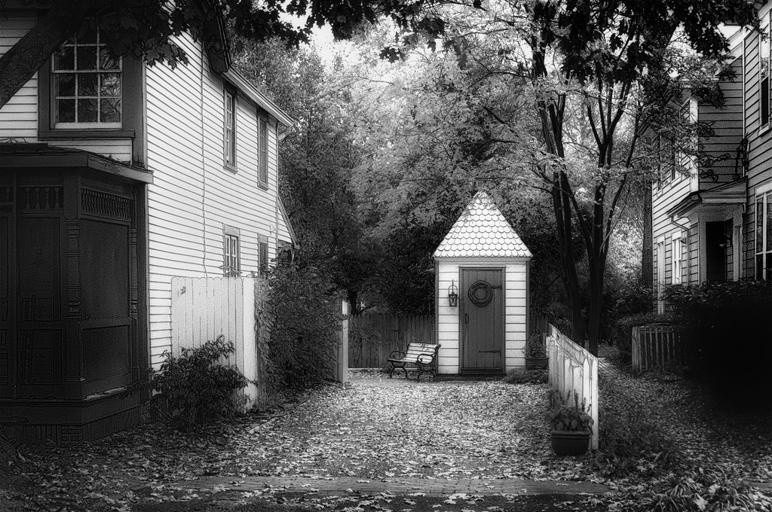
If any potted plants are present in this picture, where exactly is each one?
[546,387,593,456]
[522,332,549,370]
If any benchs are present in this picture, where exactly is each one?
[388,342,441,384]
